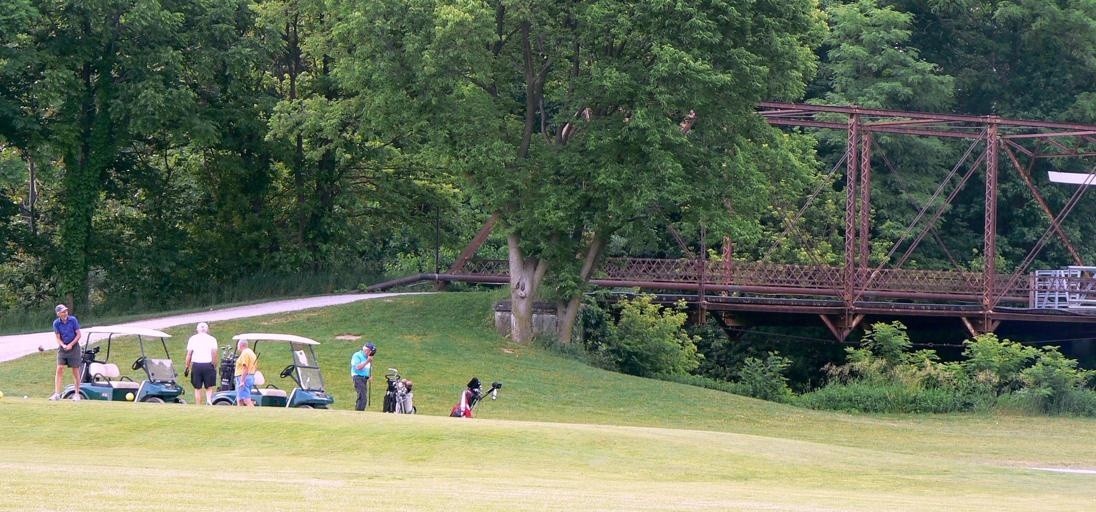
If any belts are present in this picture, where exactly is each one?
[361,376,369,379]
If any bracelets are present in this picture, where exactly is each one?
[63,344,67,350]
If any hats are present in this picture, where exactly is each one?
[197,323,208,329]
[365,342,375,350]
[55,304,69,313]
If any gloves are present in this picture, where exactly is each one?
[184,367,189,376]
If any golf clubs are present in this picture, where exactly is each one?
[176,377,188,400]
[221,344,239,360]
[82,345,99,354]
[39,347,62,352]
[224,353,261,405]
[385,368,412,394]
[369,349,376,406]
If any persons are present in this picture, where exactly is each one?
[350,342,376,411]
[182,322,219,405]
[48,304,81,401]
[233,340,257,407]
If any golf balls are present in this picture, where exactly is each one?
[24,396,27,398]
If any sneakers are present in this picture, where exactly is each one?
[206,400,212,405]
[49,393,62,400]
[72,394,80,401]
